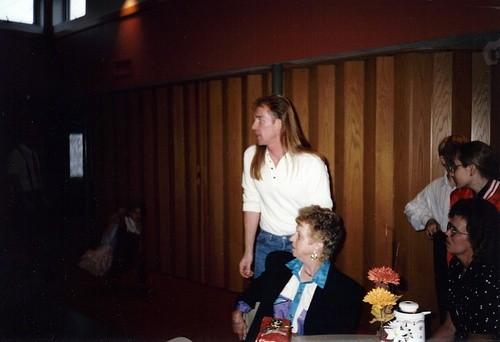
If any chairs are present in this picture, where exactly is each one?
[433,230,453,324]
[241,272,274,342]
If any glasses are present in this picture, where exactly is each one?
[449,164,469,173]
[447,221,471,237]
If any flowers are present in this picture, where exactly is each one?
[363,267,402,342]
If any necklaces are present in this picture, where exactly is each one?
[301,267,314,278]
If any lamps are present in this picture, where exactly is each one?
[69,131,86,179]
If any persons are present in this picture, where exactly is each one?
[107,202,153,285]
[403,136,499,342]
[239,95,334,281]
[232,206,369,342]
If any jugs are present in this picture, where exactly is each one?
[394,301,431,342]
